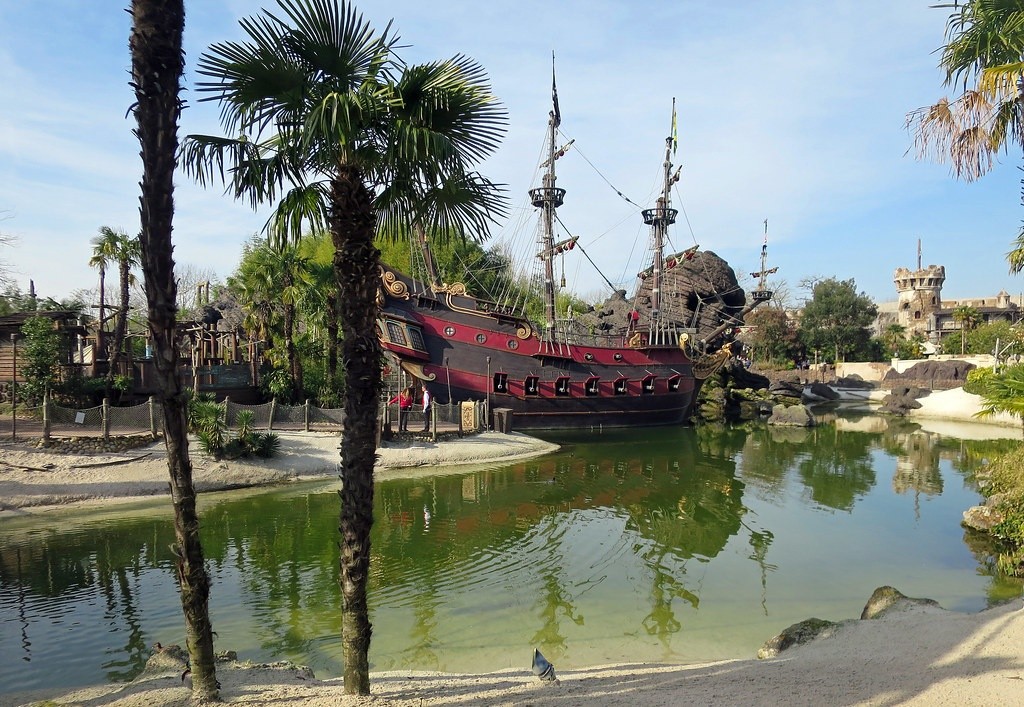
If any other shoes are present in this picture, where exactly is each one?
[421,429,428,431]
[400,429,403,431]
[404,429,408,431]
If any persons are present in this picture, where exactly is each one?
[633,310,639,325]
[627,312,632,323]
[387,387,413,431]
[421,384,430,433]
[745,359,751,369]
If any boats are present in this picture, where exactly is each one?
[375,49,779,433]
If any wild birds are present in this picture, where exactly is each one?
[151,642,182,654]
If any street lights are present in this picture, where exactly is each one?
[11,332,20,441]
